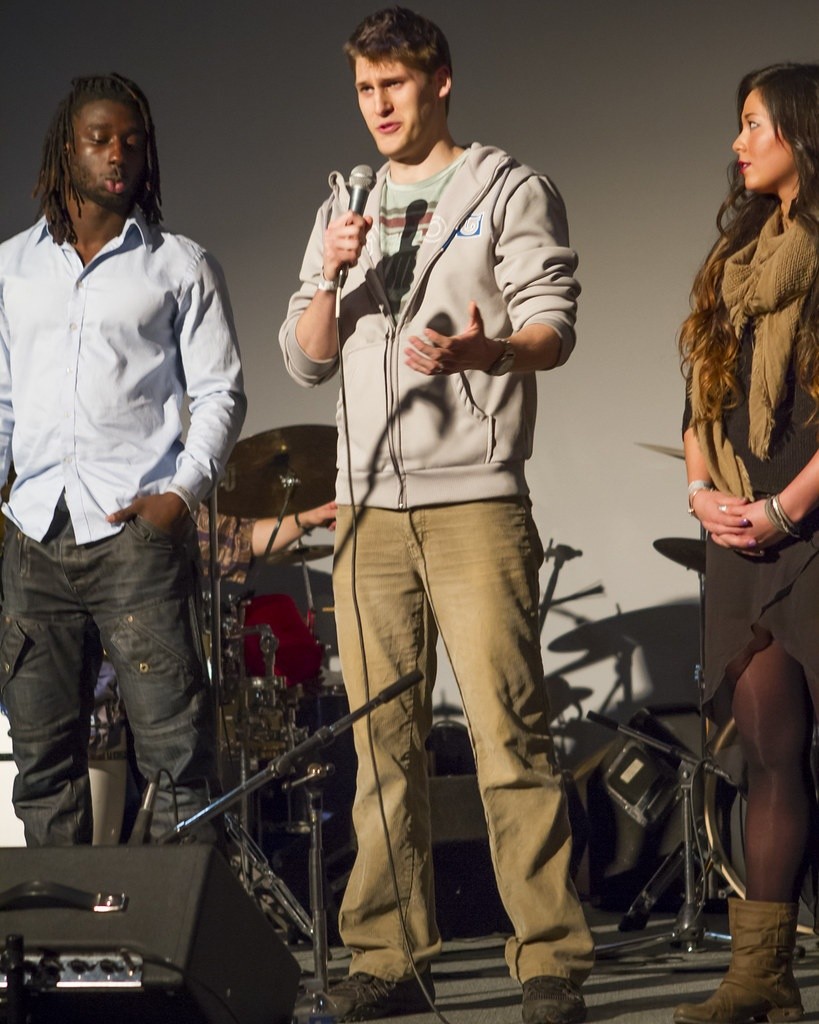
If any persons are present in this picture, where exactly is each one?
[673,62,819,1023]
[95,501,336,847]
[0,69,248,849]
[280,11,595,1024]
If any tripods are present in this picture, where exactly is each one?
[593,762,804,959]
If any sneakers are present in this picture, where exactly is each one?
[321,960,436,1022]
[521,975,587,1024]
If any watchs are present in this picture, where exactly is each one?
[484,339,516,376]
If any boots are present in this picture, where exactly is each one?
[673,896,805,1024]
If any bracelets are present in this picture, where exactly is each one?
[765,495,801,536]
[688,481,712,518]
[295,513,310,536]
[320,267,339,290]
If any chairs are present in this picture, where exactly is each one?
[244,596,321,690]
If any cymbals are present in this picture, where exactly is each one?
[653,538,707,577]
[266,544,335,566]
[202,423,339,519]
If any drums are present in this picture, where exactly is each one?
[195,589,241,755]
[296,683,357,773]
[223,675,290,764]
[701,714,819,938]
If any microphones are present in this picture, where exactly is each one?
[124,772,161,845]
[0,842,302,1024]
[334,164,376,288]
